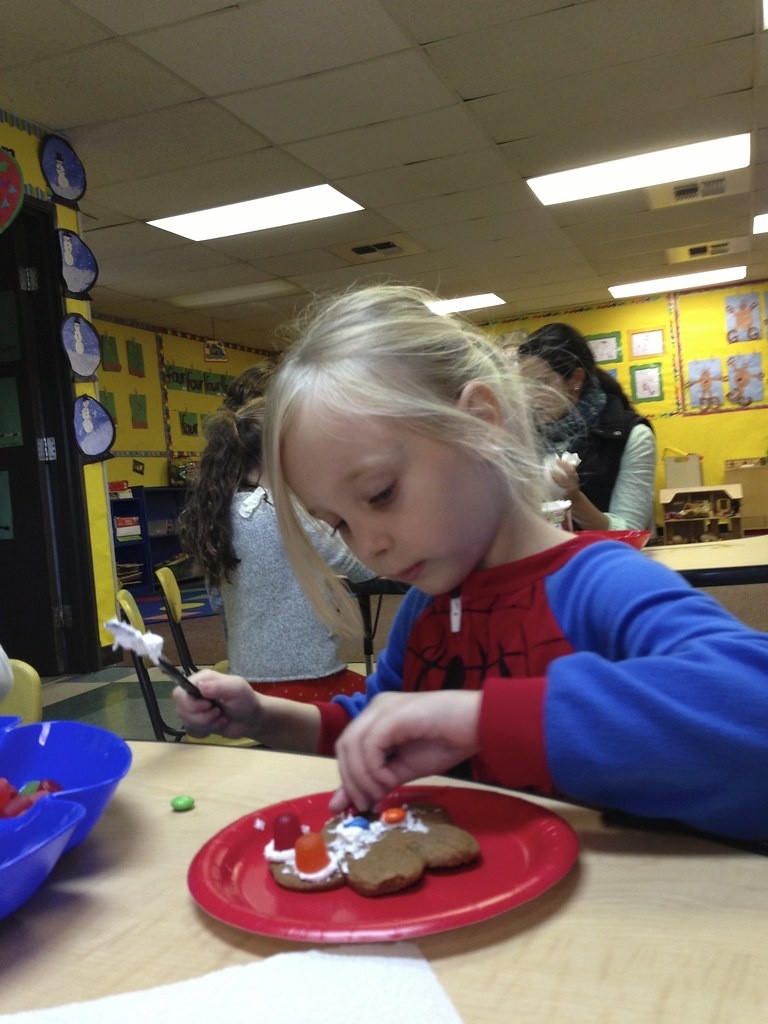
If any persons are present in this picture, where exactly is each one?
[174,355,383,714]
[507,319,661,547]
[168,269,768,857]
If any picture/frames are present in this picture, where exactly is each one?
[585,331,624,365]
[630,363,665,404]
[626,324,666,362]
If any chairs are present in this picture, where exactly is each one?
[117,590,261,749]
[153,567,229,677]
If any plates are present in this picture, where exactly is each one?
[186,783,580,945]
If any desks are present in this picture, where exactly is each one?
[0,734,768,1024]
[334,532,768,677]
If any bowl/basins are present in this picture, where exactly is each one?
[0,714,132,922]
[575,528,654,551]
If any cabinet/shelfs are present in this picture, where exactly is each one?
[110,484,200,589]
[660,484,745,545]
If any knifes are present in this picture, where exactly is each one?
[105,620,224,712]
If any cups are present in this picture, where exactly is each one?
[541,501,574,534]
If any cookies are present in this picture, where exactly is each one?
[263,802,480,897]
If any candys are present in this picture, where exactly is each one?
[0,776,64,819]
[170,795,195,810]
